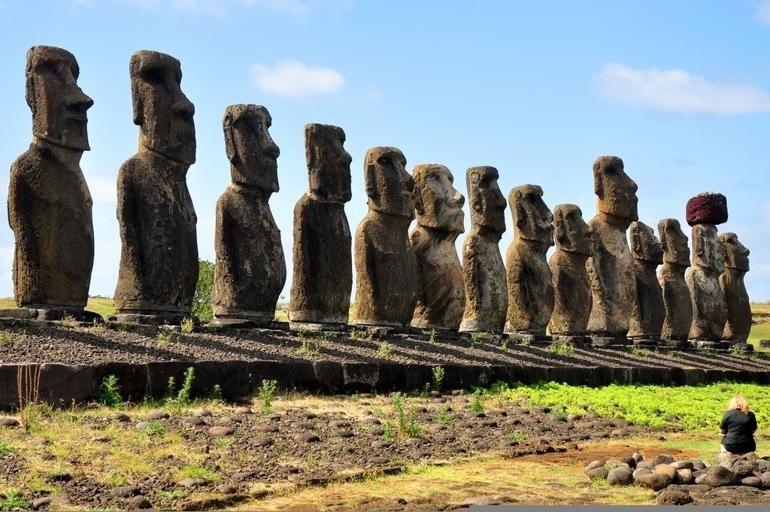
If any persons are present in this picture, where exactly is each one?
[717,397,760,456]
